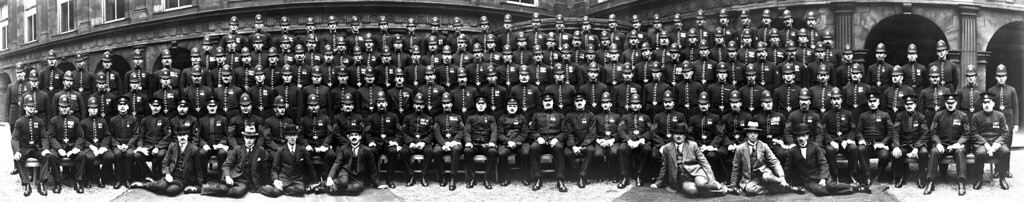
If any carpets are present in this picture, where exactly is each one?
[111,187,406,202]
[612,181,900,202]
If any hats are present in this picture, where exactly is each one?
[792,123,810,134]
[283,125,298,134]
[669,123,689,133]
[346,125,363,134]
[744,121,763,131]
[241,126,259,136]
[176,125,191,135]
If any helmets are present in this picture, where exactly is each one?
[13,10,1008,110]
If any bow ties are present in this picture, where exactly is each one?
[799,145,808,148]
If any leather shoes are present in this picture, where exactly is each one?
[10,168,1013,195]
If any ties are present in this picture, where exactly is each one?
[291,147,294,155]
[676,146,680,153]
[351,145,360,157]
[180,143,184,151]
[748,142,756,154]
[246,146,254,154]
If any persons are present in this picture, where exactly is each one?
[529,93,568,192]
[4,10,1020,201]
